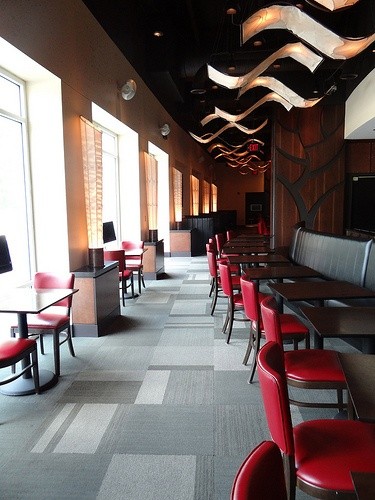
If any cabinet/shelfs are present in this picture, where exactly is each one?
[74,262,121,338]
[170,228,194,256]
[144,238,165,279]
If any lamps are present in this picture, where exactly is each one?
[119,79,138,101]
[160,123,171,137]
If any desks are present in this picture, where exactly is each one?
[0,289,79,396]
[267,279,375,350]
[240,267,320,312]
[299,307,375,349]
[339,353,375,420]
[229,256,288,292]
[117,248,148,298]
[182,210,236,252]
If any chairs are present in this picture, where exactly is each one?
[103,250,135,307]
[0,339,40,396]
[8,273,76,374]
[206,227,269,315]
[230,441,289,500]
[253,341,375,500]
[239,275,309,383]
[120,241,146,294]
[260,297,354,422]
[222,260,267,343]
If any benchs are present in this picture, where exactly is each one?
[290,228,374,308]
